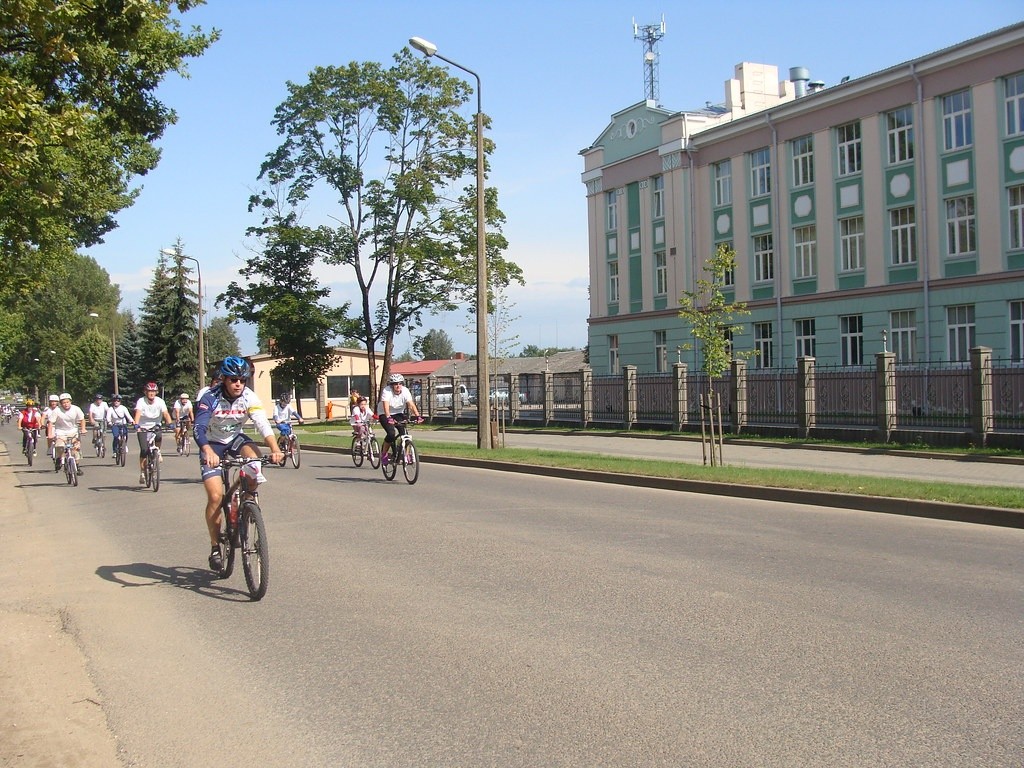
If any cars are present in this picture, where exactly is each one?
[469,387,528,407]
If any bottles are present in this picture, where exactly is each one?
[362,439,367,449]
[230,493,240,528]
[253,492,258,505]
[397,439,401,453]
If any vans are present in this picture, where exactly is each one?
[413,383,471,412]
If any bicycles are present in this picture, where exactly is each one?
[89,420,109,458]
[199,453,291,601]
[272,421,305,469]
[107,422,136,467]
[378,419,425,484]
[351,419,382,469]
[0,403,86,486]
[135,427,175,492]
[173,419,197,457]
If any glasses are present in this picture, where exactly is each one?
[393,381,402,384]
[225,376,247,384]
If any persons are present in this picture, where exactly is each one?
[172,393,195,453]
[193,356,284,572]
[0,404,15,418]
[134,382,174,485]
[349,396,379,446]
[195,370,223,409]
[47,393,87,476]
[273,393,304,448]
[89,394,109,450]
[18,399,42,456]
[40,394,61,453]
[377,373,424,466]
[106,394,135,458]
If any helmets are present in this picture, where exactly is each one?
[142,383,159,396]
[95,394,102,400]
[180,393,189,400]
[221,355,252,379]
[59,393,72,402]
[112,394,122,398]
[358,396,367,403]
[49,395,60,402]
[389,372,404,384]
[212,371,223,380]
[26,400,35,405]
[280,393,291,403]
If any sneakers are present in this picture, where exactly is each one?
[211,549,222,569]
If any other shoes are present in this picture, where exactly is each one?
[140,474,145,485]
[159,454,164,464]
[21,436,129,476]
[381,450,388,466]
[407,455,413,464]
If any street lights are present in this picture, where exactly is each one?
[408,34,493,451]
[50,350,66,392]
[89,312,119,395]
[159,248,206,389]
